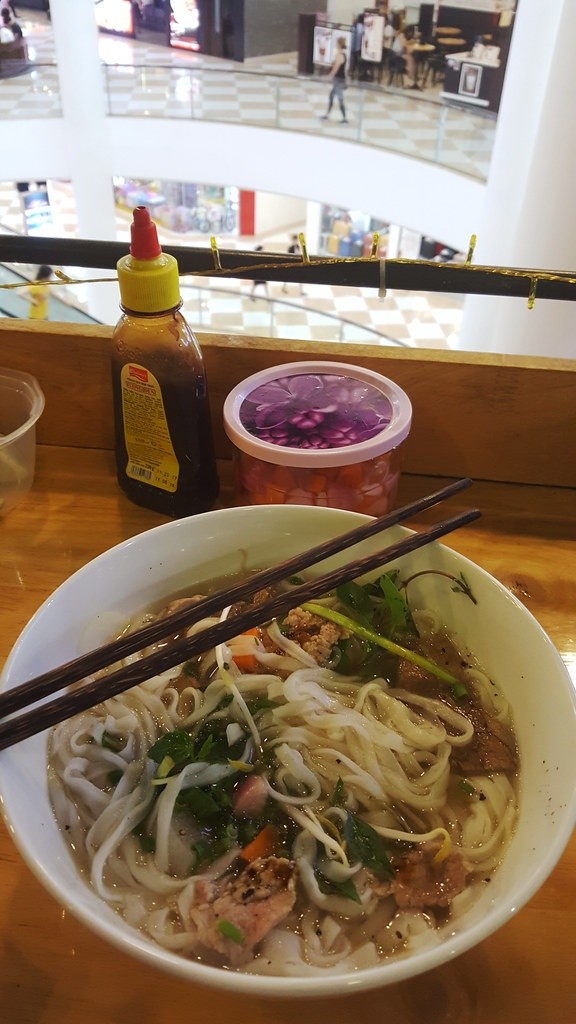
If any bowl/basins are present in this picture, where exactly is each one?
[0,504,576,998]
[223,360,414,518]
[0,366,45,518]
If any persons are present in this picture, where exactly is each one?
[319,37,349,124]
[282,235,308,295]
[17,266,53,320]
[249,245,272,302]
[353,8,416,82]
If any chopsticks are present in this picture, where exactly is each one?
[0,476,483,752]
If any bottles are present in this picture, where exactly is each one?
[110,205,220,519]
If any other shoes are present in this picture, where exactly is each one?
[321,117,327,119]
[340,120,348,123]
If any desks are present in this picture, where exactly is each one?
[438,37,464,51]
[436,25,461,37]
[0,441,576,1024]
[412,42,436,67]
